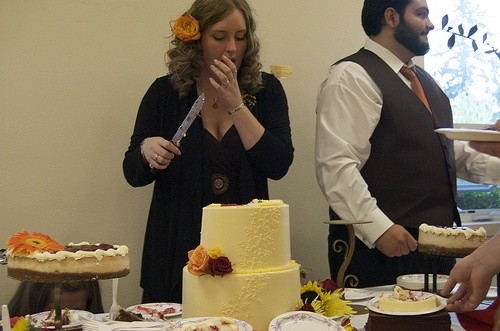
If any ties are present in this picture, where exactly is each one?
[399,67,432,115]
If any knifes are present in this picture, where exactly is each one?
[149,90,206,172]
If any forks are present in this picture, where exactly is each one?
[109,279,119,321]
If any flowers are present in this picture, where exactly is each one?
[11,315,34,331]
[292,266,357,331]
[187,246,233,279]
[6,230,63,257]
[171,12,202,41]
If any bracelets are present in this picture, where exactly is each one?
[226,102,244,115]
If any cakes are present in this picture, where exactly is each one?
[135,305,175,315]
[6,230,130,282]
[182,199,304,331]
[377,285,436,313]
[417,223,487,258]
[40,308,72,325]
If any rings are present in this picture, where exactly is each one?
[155,154,160,161]
[222,80,229,85]
[149,163,153,168]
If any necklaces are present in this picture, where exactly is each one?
[197,84,225,108]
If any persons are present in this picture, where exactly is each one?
[122,0,295,304]
[315,0,500,313]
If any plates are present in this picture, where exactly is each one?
[0,300,349,331]
[342,288,379,301]
[455,285,498,309]
[396,274,449,290]
[433,127,500,143]
[367,290,448,316]
